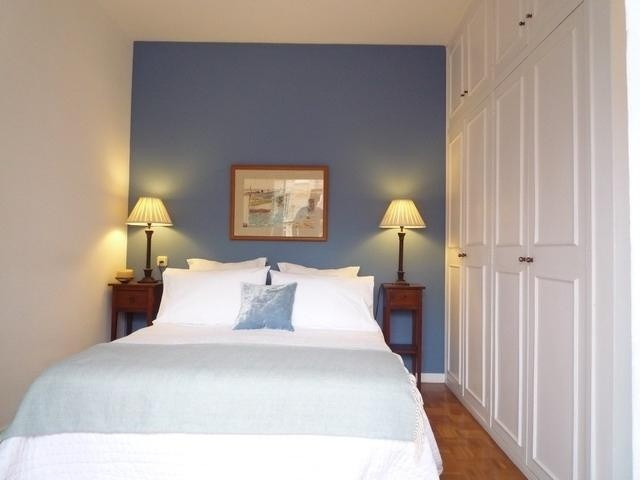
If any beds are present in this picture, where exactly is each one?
[0,326,443,480]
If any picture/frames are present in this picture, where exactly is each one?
[229,164,330,241]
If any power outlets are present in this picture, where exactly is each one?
[157,256,168,267]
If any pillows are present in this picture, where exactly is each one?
[186,258,266,270]
[270,270,380,330]
[277,262,361,274]
[152,266,270,328]
[232,281,297,332]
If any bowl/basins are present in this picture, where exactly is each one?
[115,277,134,284]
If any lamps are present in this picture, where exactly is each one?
[125,197,173,283]
[379,199,426,285]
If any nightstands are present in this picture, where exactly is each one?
[382,282,426,392]
[108,283,163,341]
